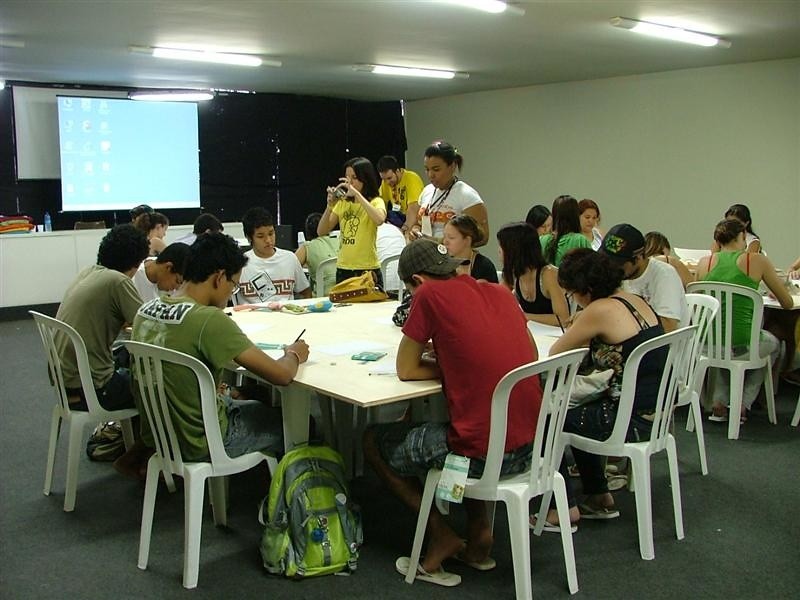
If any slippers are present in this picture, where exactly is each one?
[568,463,619,476]
[606,475,628,490]
[740,417,746,423]
[578,503,620,519]
[396,556,461,587]
[451,538,497,570]
[528,513,578,533]
[709,408,728,421]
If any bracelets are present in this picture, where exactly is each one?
[285,349,300,365]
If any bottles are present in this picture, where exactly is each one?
[44,212,51,232]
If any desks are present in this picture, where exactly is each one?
[0,221,249,320]
[760,278,800,312]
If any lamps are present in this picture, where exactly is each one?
[126,44,282,69]
[349,61,469,82]
[124,88,217,103]
[432,0,526,18]
[608,16,733,50]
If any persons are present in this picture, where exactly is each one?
[47,141,799,586]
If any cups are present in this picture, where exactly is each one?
[37,225,44,232]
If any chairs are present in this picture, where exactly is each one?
[117,336,279,589]
[683,279,778,442]
[404,345,591,600]
[27,308,177,514]
[75,221,106,229]
[311,255,338,297]
[531,323,702,561]
[381,255,407,297]
[628,292,723,491]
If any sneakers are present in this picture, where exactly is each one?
[114,450,146,480]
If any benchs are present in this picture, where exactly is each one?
[212,294,564,463]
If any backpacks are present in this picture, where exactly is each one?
[259,445,364,577]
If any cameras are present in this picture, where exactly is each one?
[334,188,346,199]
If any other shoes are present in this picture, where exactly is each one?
[640,411,664,422]
[784,378,800,386]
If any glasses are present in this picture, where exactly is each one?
[229,279,241,295]
[565,288,579,304]
[345,174,357,180]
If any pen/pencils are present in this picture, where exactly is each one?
[556,315,564,333]
[368,372,397,376]
[295,329,306,343]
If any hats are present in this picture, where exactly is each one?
[398,238,464,281]
[129,205,155,218]
[597,224,647,268]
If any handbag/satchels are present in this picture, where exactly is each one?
[548,369,614,415]
[86,420,125,462]
